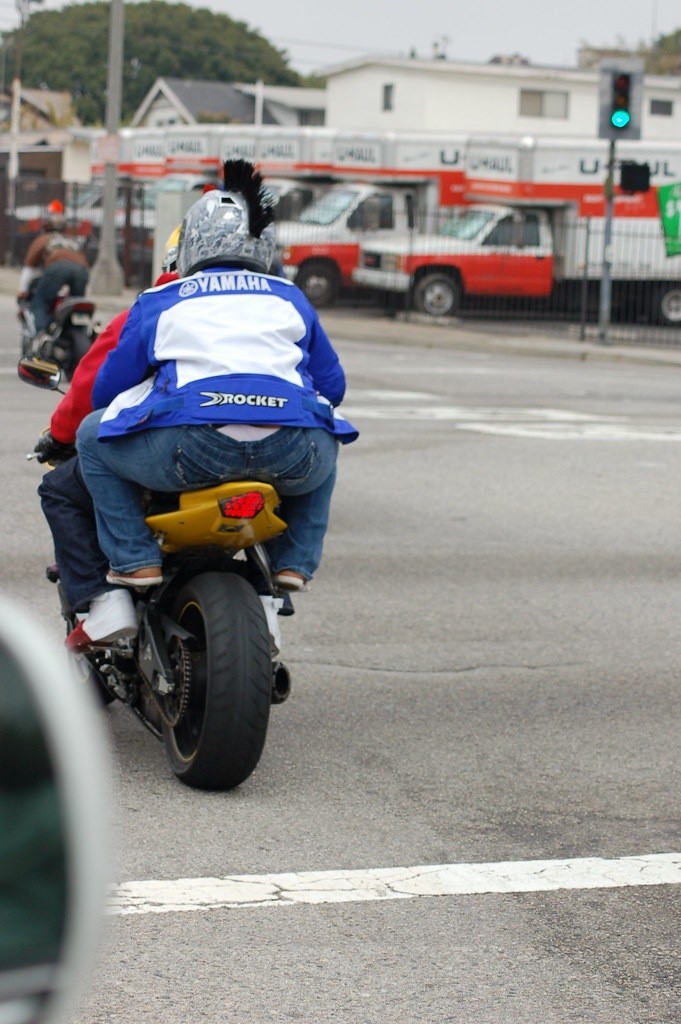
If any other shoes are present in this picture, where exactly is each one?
[274,570,308,588]
[105,563,165,586]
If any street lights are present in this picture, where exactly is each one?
[4,0,54,255]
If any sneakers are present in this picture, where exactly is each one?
[66,588,137,653]
[259,594,283,655]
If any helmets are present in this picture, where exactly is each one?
[45,214,67,231]
[176,157,279,273]
[159,222,181,274]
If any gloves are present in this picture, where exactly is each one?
[36,433,76,467]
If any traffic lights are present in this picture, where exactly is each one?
[599,57,645,141]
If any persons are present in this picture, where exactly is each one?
[31,180,361,655]
[16,213,91,353]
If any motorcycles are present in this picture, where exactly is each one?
[16,277,106,385]
[16,355,294,793]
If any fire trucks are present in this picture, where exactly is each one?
[261,135,476,311]
[0,127,289,298]
[355,135,681,329]
